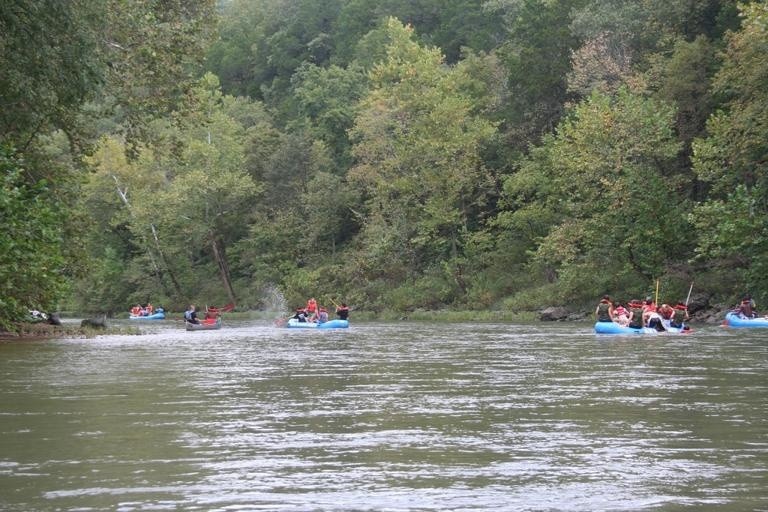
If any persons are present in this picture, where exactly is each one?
[288,296,328,323]
[594,290,694,334]
[127,301,165,315]
[184,305,196,322]
[741,294,757,309]
[336,303,349,320]
[731,297,752,317]
[188,313,199,325]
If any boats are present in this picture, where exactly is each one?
[287,317,349,328]
[594,316,691,339]
[186,317,222,330]
[128,308,166,320]
[722,309,767,331]
